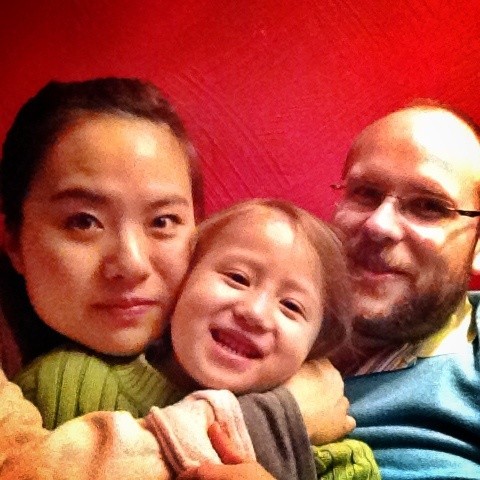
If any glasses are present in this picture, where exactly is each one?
[327,183,479,224]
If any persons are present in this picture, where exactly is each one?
[325,106,480,480]
[7,197,384,479]
[1,76,356,480]
